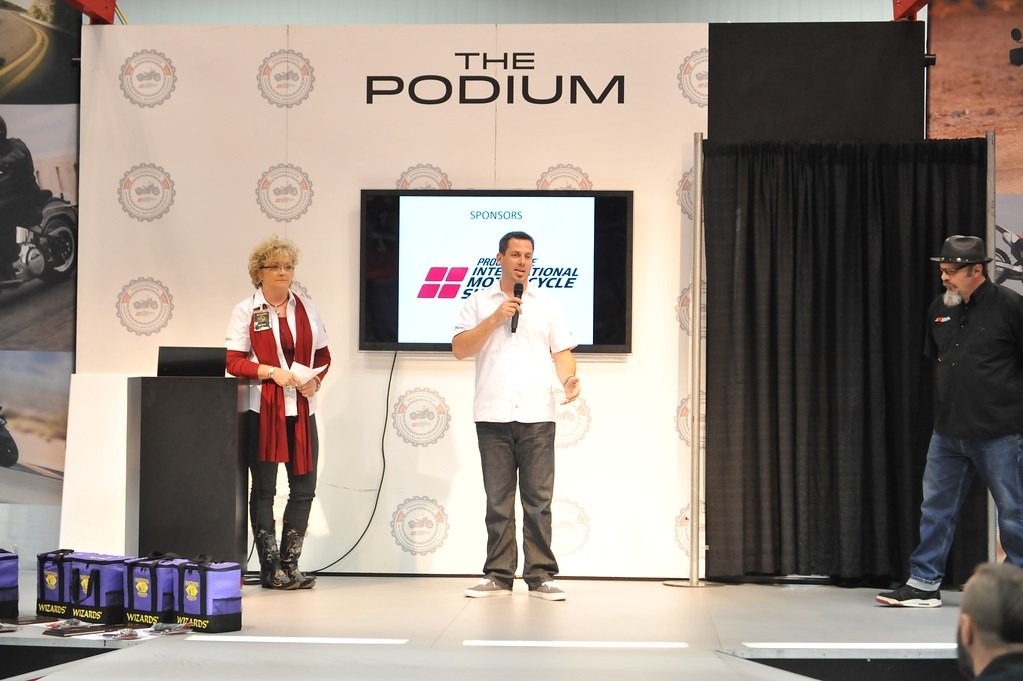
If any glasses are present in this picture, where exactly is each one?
[938,263,969,275]
[259,264,295,270]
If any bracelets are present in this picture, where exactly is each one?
[563,376,575,388]
[268,366,276,378]
[313,377,321,392]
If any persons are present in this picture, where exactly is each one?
[876,235,1023,608]
[0,116,43,282]
[225,238,331,590]
[452,232,580,601]
[956,562,1023,681]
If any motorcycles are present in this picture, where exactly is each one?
[992,224,1023,284]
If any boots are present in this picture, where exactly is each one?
[277,529,317,589]
[253,519,301,590]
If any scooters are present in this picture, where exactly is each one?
[0,197,80,293]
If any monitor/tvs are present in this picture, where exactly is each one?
[358,189,634,356]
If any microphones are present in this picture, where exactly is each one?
[511,283,523,333]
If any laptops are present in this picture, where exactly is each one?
[157,346,226,377]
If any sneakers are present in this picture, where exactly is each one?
[463,577,513,597]
[527,580,567,601]
[875,584,942,608]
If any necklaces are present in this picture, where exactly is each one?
[264,296,288,315]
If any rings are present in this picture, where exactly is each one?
[287,386,290,388]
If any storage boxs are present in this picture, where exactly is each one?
[0,548,242,634]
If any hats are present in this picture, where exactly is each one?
[930,235,993,264]
